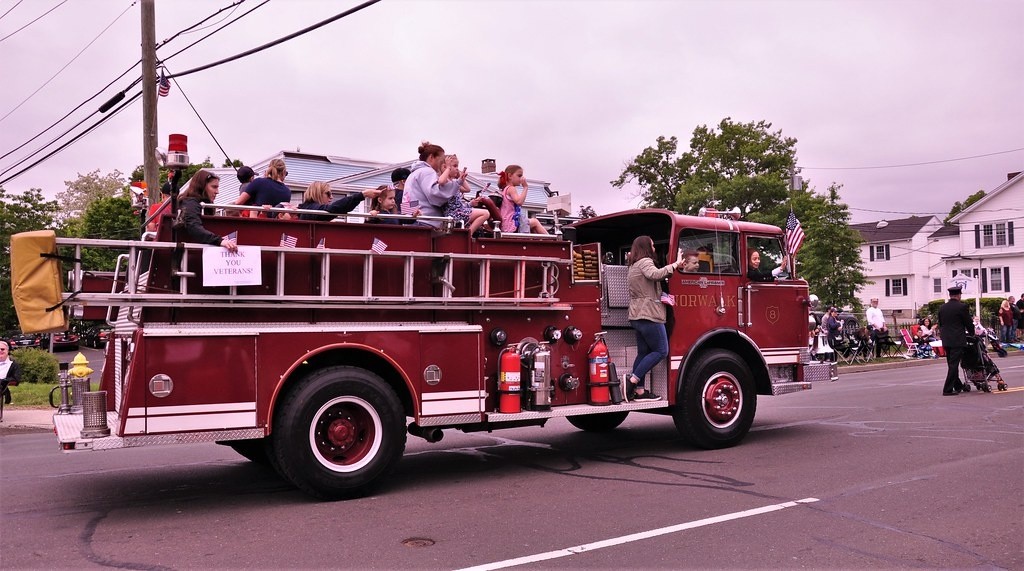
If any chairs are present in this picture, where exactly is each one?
[829,328,921,366]
[697,254,714,273]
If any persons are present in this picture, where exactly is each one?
[809,306,851,358]
[178,169,238,253]
[364,184,420,225]
[218,158,291,219]
[999,293,1024,342]
[298,180,382,221]
[391,141,549,237]
[0,338,17,397]
[748,247,787,281]
[857,297,888,360]
[145,182,180,240]
[273,201,300,219]
[623,237,685,403]
[937,287,978,396]
[973,316,987,334]
[237,166,259,205]
[913,317,939,359]
[681,249,699,272]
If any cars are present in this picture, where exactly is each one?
[84,324,113,350]
[0,330,80,354]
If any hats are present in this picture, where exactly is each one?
[391,167,411,182]
[830,307,839,312]
[947,287,962,295]
[237,166,259,179]
[871,298,879,302]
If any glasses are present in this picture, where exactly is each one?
[322,191,332,198]
[284,169,288,176]
[206,173,217,180]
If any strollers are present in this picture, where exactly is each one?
[960,333,1008,393]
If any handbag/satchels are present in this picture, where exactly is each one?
[662,280,675,339]
[831,327,872,348]
[518,209,530,233]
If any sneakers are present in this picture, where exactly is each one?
[623,373,637,403]
[634,389,661,402]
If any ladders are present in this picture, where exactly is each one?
[12,231,571,303]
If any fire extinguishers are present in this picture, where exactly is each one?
[527,340,553,409]
[587,331,610,402]
[498,343,520,413]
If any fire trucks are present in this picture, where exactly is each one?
[10,131,842,503]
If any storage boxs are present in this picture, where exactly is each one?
[930,341,947,356]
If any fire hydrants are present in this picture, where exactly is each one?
[67,352,94,400]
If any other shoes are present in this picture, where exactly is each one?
[1000,339,1024,344]
[956,385,967,392]
[876,355,881,358]
[943,391,958,396]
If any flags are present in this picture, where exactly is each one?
[785,209,806,257]
